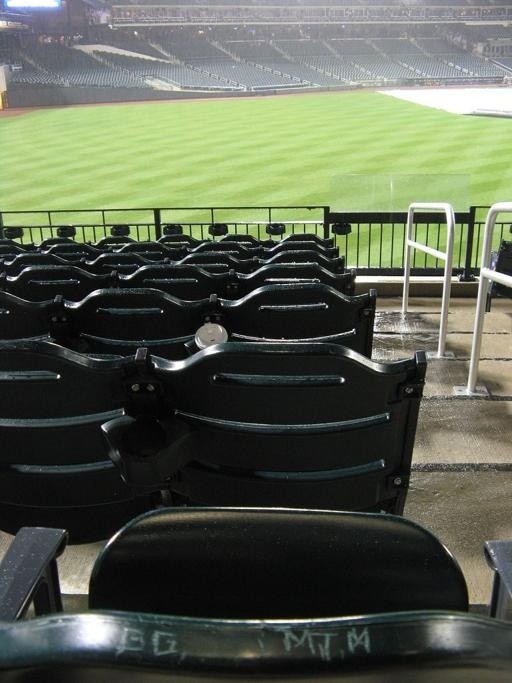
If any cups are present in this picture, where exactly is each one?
[194,320,228,350]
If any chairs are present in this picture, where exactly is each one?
[1,226,512,682]
[0,16,512,93]
